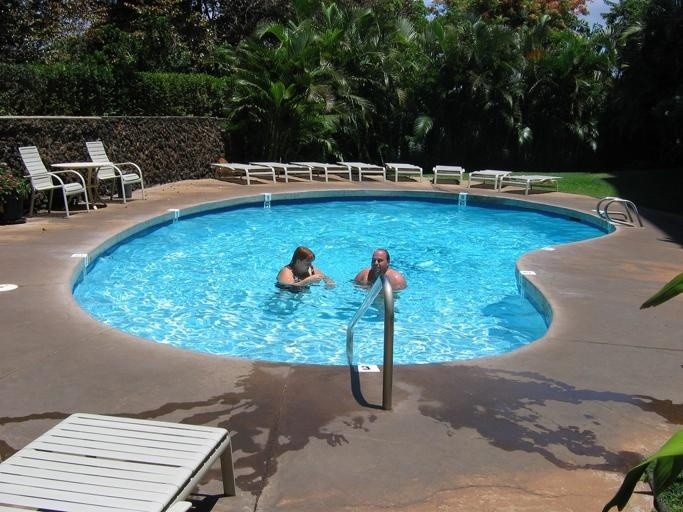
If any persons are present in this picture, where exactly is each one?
[276,246,336,290]
[354,249,407,290]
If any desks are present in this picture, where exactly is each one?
[50,162,126,209]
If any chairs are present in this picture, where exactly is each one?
[0,412,236,512]
[18,145,90,218]
[468,170,561,195]
[432,165,465,184]
[86,141,145,203]
[210,162,423,186]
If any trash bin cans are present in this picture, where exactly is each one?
[117,173,132,198]
